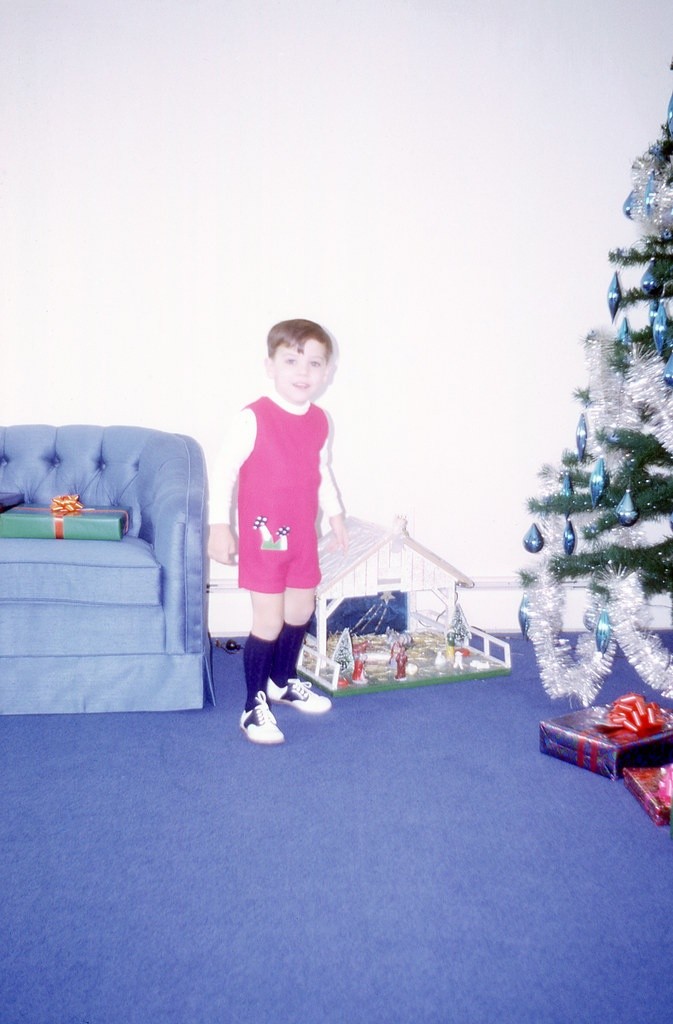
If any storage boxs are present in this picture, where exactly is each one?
[622,767,673,827]
[539,704,673,781]
[0,505,132,541]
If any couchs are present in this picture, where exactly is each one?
[0,424,217,716]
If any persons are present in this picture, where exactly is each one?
[206,318,348,745]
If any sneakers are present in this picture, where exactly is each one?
[267,680,332,713]
[240,690,284,744]
